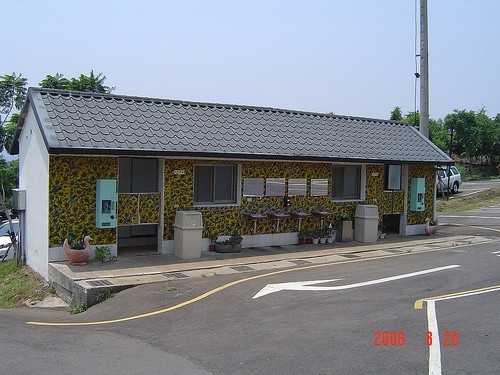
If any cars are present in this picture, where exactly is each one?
[0,221,21,260]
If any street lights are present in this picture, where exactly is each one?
[447,125,456,201]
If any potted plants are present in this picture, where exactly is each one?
[216,236,243,253]
[425,217,438,235]
[63,235,93,266]
[378,224,386,239]
[209,232,217,251]
[298,228,334,245]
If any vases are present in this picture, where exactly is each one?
[337,221,353,243]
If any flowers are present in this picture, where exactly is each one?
[337,213,350,221]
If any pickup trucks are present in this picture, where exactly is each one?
[433,166,463,192]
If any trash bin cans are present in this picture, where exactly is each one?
[354,204,379,243]
[172,210,203,261]
